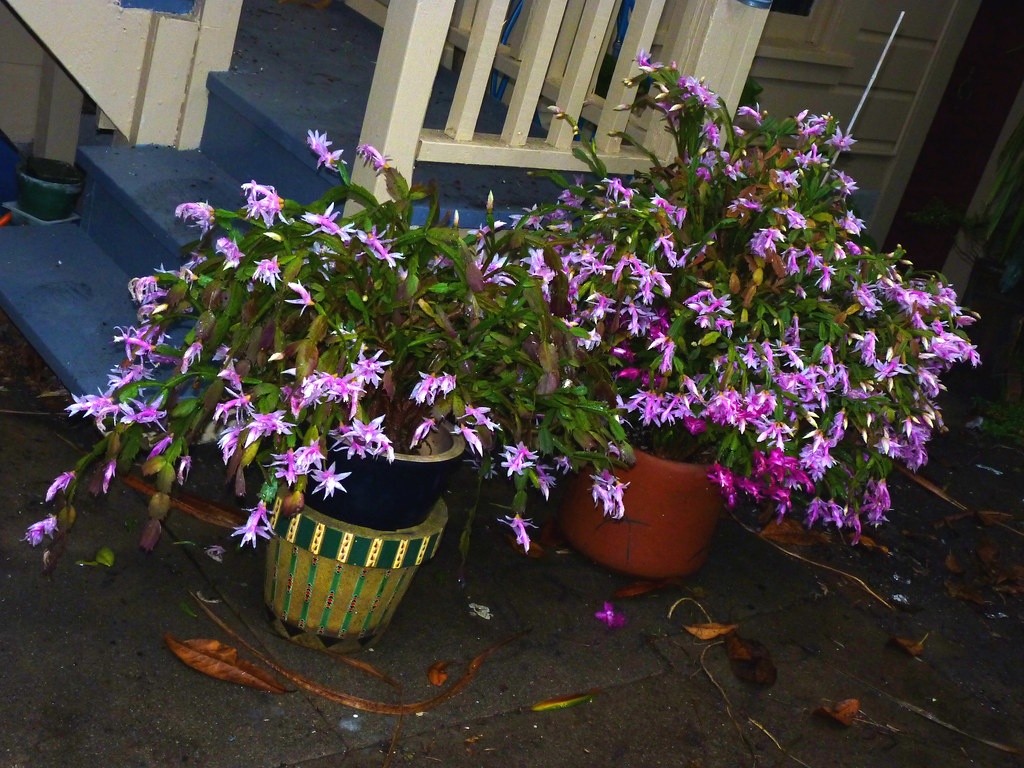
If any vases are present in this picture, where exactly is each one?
[265,416,473,643]
[1,171,83,227]
[563,446,731,590]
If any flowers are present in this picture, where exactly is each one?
[16,52,984,558]
